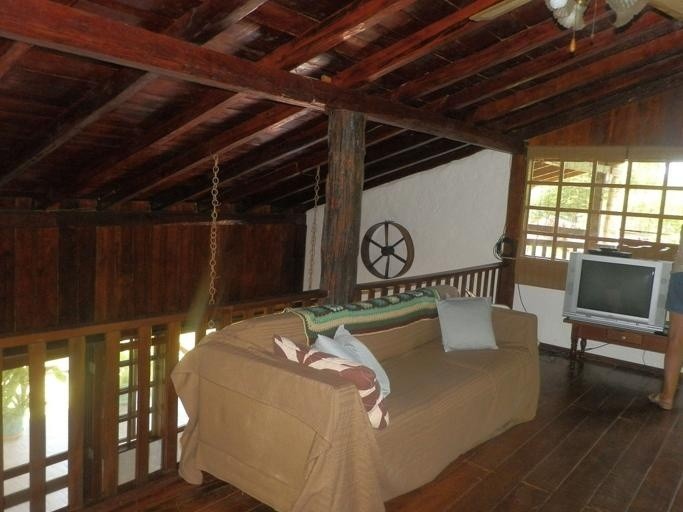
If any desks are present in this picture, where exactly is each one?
[564,319,668,359]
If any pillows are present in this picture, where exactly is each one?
[266,321,394,428]
[435,293,500,352]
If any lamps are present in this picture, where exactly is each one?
[543,0,649,55]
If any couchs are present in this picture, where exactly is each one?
[169,284,538,512]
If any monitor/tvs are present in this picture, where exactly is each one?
[562,252,674,334]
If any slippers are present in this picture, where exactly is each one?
[648,391,674,411]
